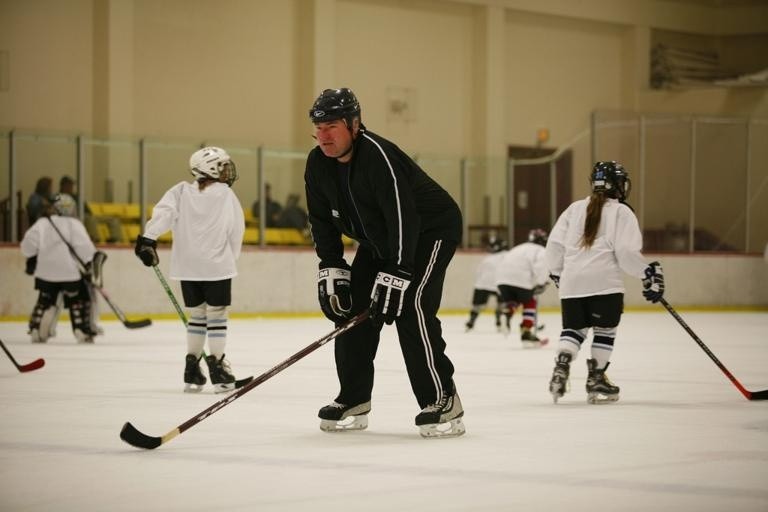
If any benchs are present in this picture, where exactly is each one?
[92,223,359,246]
[84,201,281,228]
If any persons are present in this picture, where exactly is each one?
[545,160,666,406]
[59,176,91,224]
[281,193,308,228]
[27,178,53,226]
[304,87,463,425]
[253,184,283,228]
[20,195,98,343]
[140,148,247,384]
[497,228,552,340]
[466,250,511,328]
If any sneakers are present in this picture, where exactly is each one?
[185,355,207,384]
[74,327,97,340]
[550,362,569,398]
[522,331,539,342]
[318,395,371,422]
[205,354,235,383]
[586,359,620,393]
[415,390,464,425]
[29,326,51,343]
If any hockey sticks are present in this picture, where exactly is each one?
[535,293,544,330]
[0,341,45,372]
[661,299,767,400]
[42,209,151,328]
[120,311,370,448]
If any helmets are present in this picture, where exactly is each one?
[309,87,360,122]
[590,160,632,200]
[492,240,508,252]
[190,146,237,186]
[529,228,547,242]
[53,193,78,216]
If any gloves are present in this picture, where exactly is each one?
[26,256,36,275]
[370,265,413,325]
[317,267,352,325]
[642,261,665,304]
[134,234,159,266]
